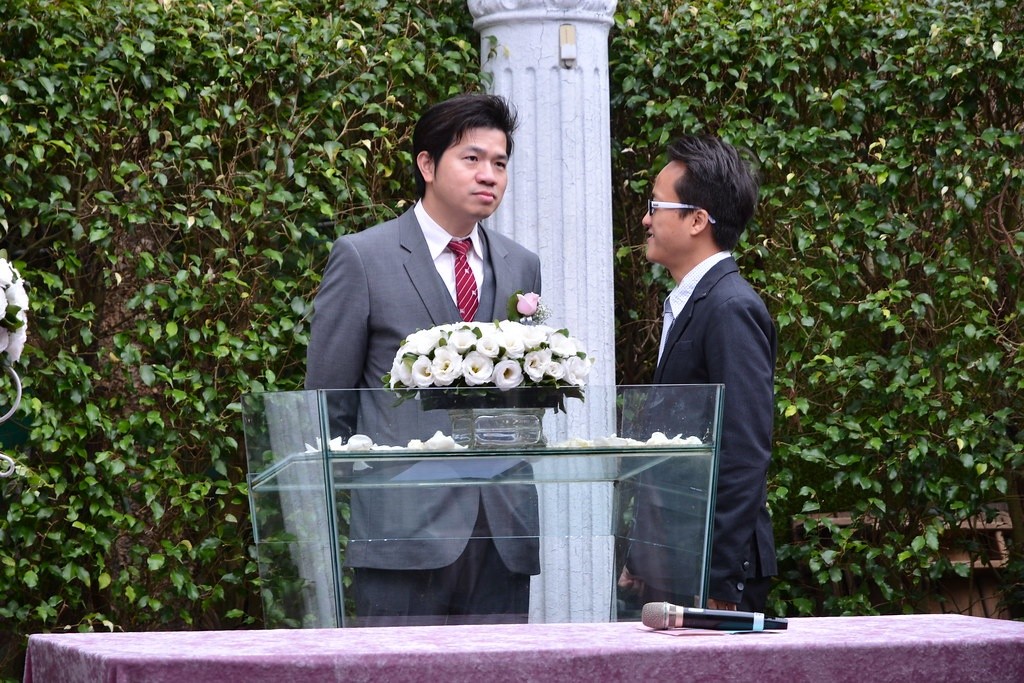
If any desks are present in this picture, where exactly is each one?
[23,612,1024,682]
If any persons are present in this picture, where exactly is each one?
[303,94,543,628]
[617,135,779,613]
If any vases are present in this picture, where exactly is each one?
[448,408,548,452]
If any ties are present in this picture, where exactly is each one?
[657,297,675,369]
[446,237,479,322]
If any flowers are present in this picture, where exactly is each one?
[384,290,593,414]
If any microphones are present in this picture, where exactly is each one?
[642,602,788,632]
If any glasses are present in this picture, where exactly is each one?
[648,199,716,224]
